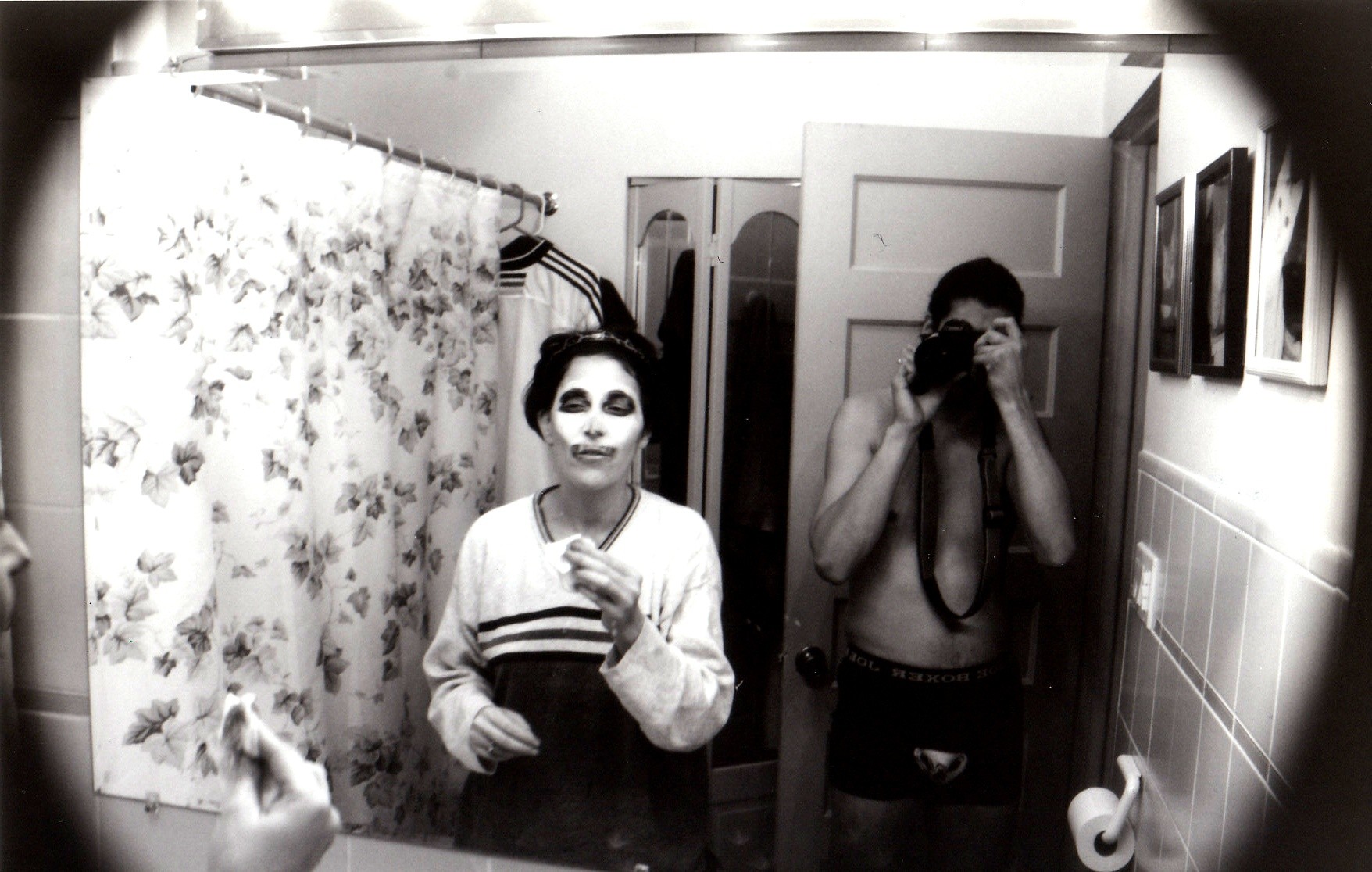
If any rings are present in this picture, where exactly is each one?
[485,741,497,753]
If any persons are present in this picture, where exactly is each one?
[424,323,738,870]
[0,487,343,872]
[813,256,1089,870]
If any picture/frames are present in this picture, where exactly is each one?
[1152,111,1335,386]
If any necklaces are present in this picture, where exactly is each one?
[534,482,640,557]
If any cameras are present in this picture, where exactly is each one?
[913,328,997,381]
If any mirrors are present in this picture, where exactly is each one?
[83,53,1346,867]
